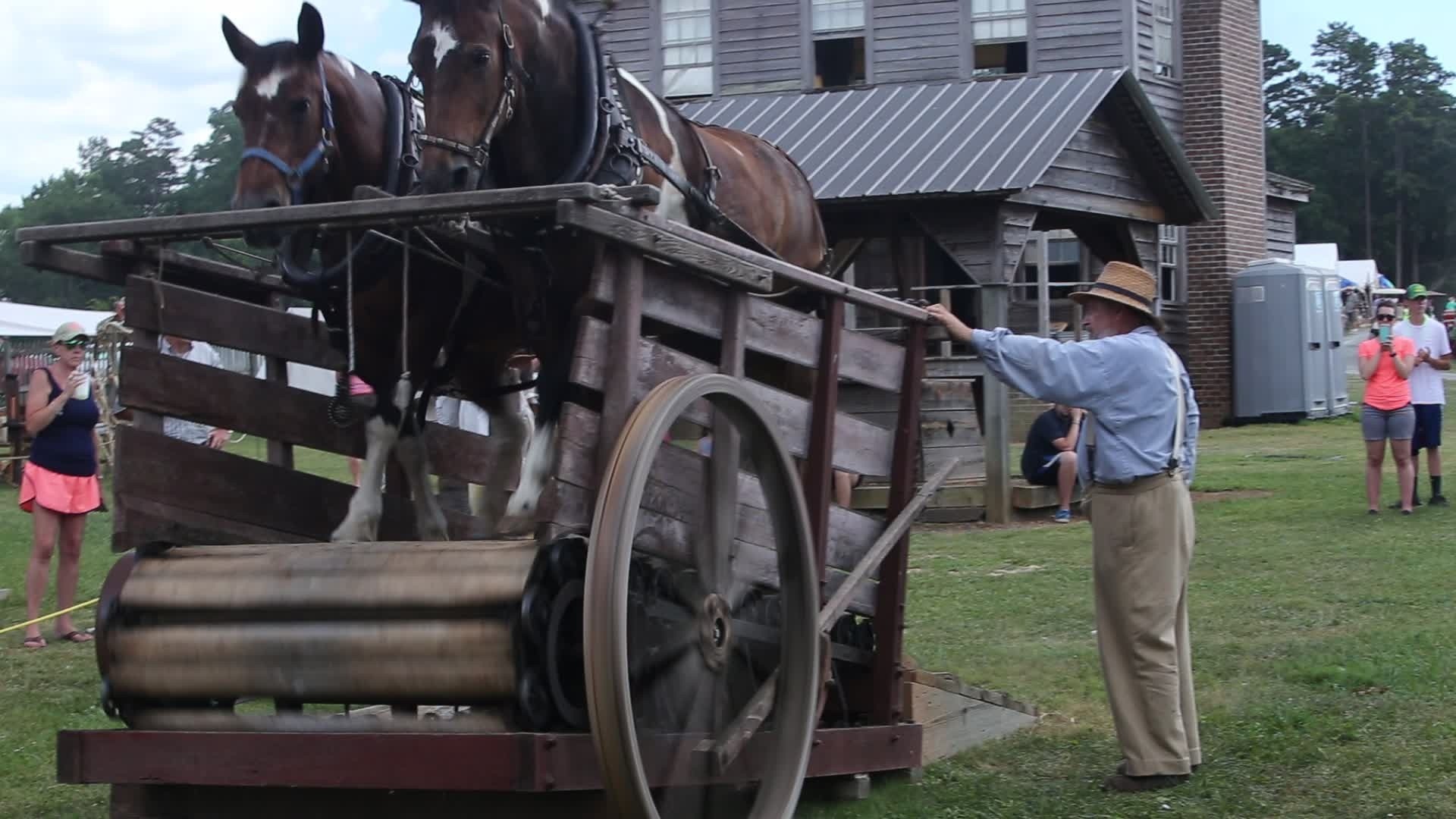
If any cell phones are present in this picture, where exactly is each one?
[1379,323,1390,344]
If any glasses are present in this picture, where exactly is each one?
[1375,313,1397,321]
[59,339,86,351]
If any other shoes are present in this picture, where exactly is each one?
[1370,509,1378,515]
[1429,494,1447,505]
[1412,493,1423,507]
[1104,772,1187,793]
[1402,509,1414,516]
[1117,762,1200,775]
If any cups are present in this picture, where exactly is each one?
[72,374,89,400]
[1379,324,1391,344]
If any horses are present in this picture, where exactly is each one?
[222,4,535,544]
[408,0,832,536]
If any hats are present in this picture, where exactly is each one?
[52,321,89,346]
[1406,284,1427,300]
[1068,262,1166,335]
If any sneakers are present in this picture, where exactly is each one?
[1055,508,1070,523]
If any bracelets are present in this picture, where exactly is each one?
[1390,353,1396,357]
[1072,422,1080,425]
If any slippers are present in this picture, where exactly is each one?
[25,635,47,649]
[62,630,86,642]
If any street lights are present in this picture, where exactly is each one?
[1361,90,1423,258]
[1395,105,1456,289]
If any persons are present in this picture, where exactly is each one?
[1021,404,1088,522]
[97,301,133,405]
[1390,284,1451,510]
[927,261,1202,790]
[158,334,230,449]
[831,471,863,508]
[1359,301,1415,514]
[1445,297,1456,309]
[18,322,110,647]
[349,375,374,485]
